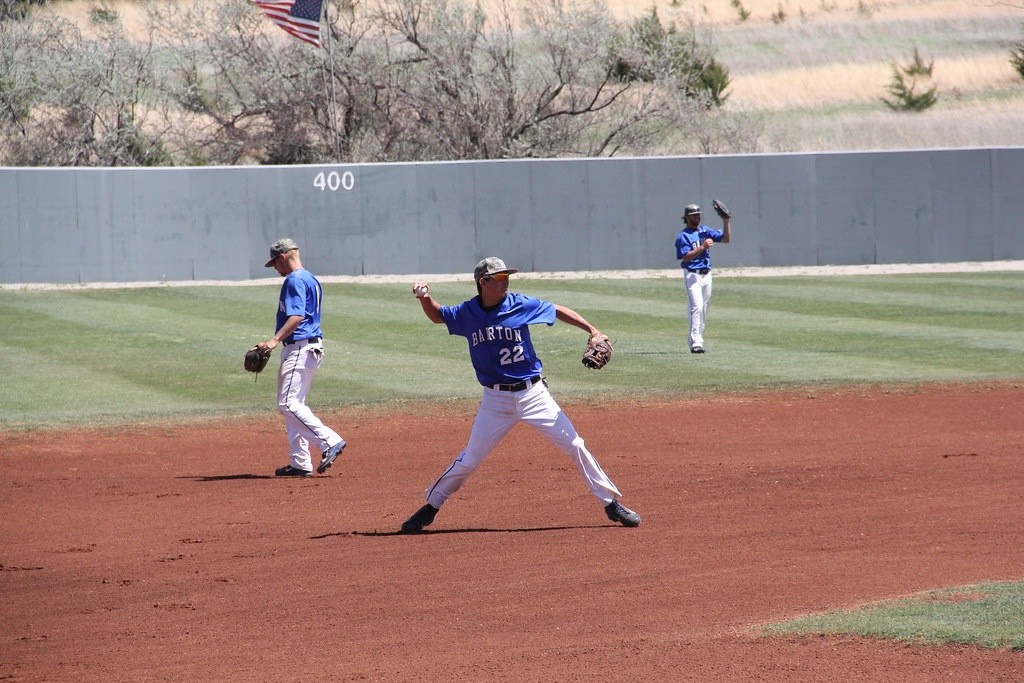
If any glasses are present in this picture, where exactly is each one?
[273,257,280,264]
[479,274,509,285]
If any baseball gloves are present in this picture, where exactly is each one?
[712,199,732,219]
[243,341,272,382]
[580,332,618,371]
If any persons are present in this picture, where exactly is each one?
[259,238,347,477]
[400,256,641,531]
[675,200,731,353]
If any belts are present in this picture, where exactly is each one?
[287,337,319,343]
[487,376,540,392]
[689,269,708,274]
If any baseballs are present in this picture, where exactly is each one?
[416,286,427,296]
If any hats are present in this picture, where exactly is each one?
[685,204,702,215]
[265,238,299,267]
[474,257,519,284]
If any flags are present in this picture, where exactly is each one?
[255,0,324,49]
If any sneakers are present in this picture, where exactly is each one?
[691,346,705,353]
[275,465,313,477]
[605,499,641,527]
[401,505,436,531]
[317,440,347,474]
